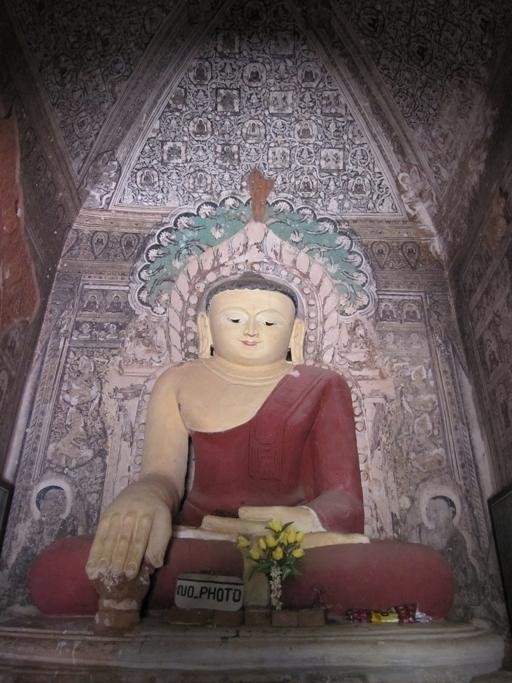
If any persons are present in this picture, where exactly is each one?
[106,295,122,313]
[381,303,396,321]
[404,304,421,322]
[27,269,455,624]
[0,485,80,617]
[415,494,493,631]
[80,296,99,312]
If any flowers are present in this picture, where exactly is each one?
[237,520,305,610]
